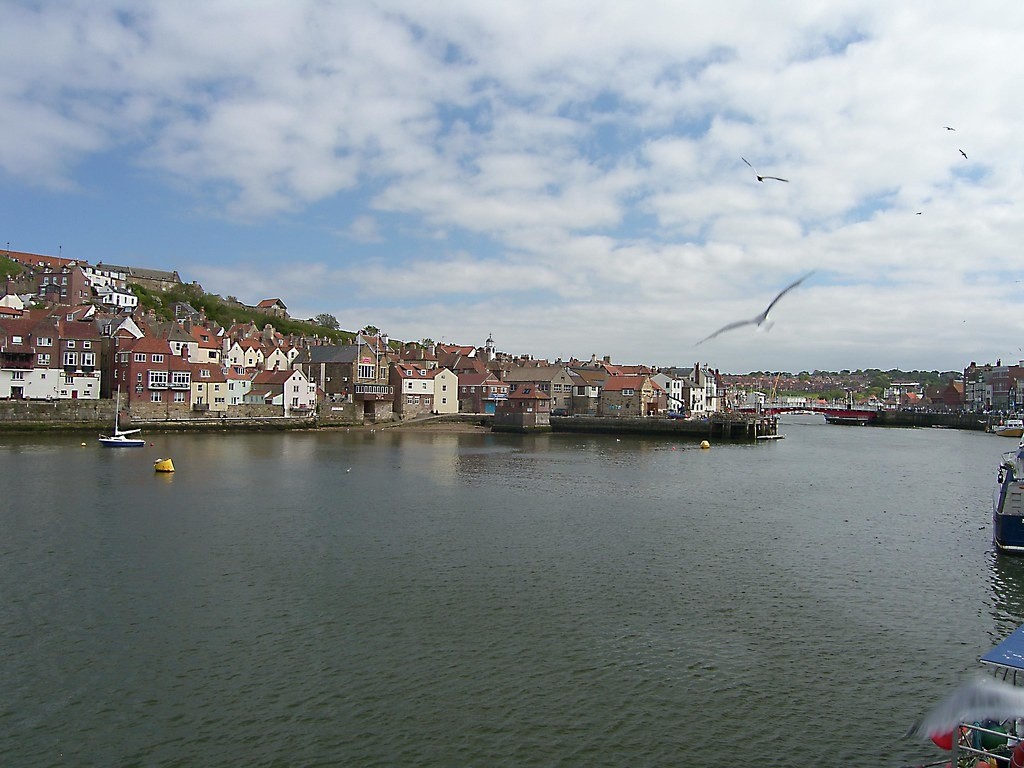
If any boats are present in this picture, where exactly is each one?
[930,623,1024,766]
[985,414,1024,437]
[993,432,1024,554]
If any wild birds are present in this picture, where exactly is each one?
[941,125,958,133]
[914,210,925,217]
[692,265,826,351]
[738,152,790,185]
[958,148,969,163]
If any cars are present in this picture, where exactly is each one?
[551,407,569,417]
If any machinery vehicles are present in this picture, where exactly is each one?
[662,392,690,419]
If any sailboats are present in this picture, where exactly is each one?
[98,384,146,447]
